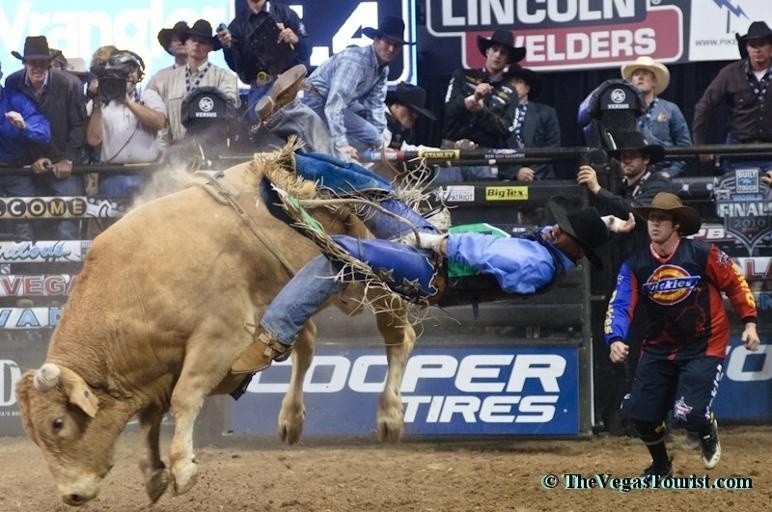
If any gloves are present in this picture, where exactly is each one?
[404,231,450,255]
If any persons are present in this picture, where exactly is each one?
[0,0,772,275]
[231,64,637,374]
[604,192,761,484]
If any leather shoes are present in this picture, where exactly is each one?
[255,64,307,123]
[230,332,292,375]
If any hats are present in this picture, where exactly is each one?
[158,22,189,54]
[385,81,437,121]
[735,20,772,58]
[362,15,418,46]
[549,131,703,273]
[476,30,526,64]
[621,55,671,95]
[503,62,541,99]
[175,19,223,52]
[11,35,62,62]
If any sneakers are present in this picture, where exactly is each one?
[636,462,674,484]
[699,416,721,471]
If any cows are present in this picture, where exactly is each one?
[11,149,421,510]
